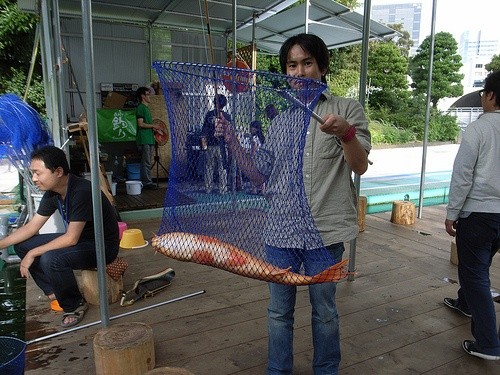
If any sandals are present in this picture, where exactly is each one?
[36,292,57,302]
[61,298,89,327]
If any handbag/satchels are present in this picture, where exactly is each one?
[119,268,175,306]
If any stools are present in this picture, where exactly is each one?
[450,240,458,266]
[390,201,416,225]
[80,268,124,306]
[93,322,155,375]
[357,195,367,232]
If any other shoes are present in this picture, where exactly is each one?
[204,188,258,194]
[463,340,500,361]
[445,297,476,316]
[143,184,156,189]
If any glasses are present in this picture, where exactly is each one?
[479,88,493,96]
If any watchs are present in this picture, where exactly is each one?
[339,124,356,142]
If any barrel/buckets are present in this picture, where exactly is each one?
[126,181,143,195]
[118,221,149,249]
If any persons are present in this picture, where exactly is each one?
[263,104,279,122]
[213,32,372,374]
[201,93,232,195]
[443,72,499,360]
[248,120,266,195]
[136,87,162,191]
[0,145,121,327]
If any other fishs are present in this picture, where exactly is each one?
[151,232,358,286]
